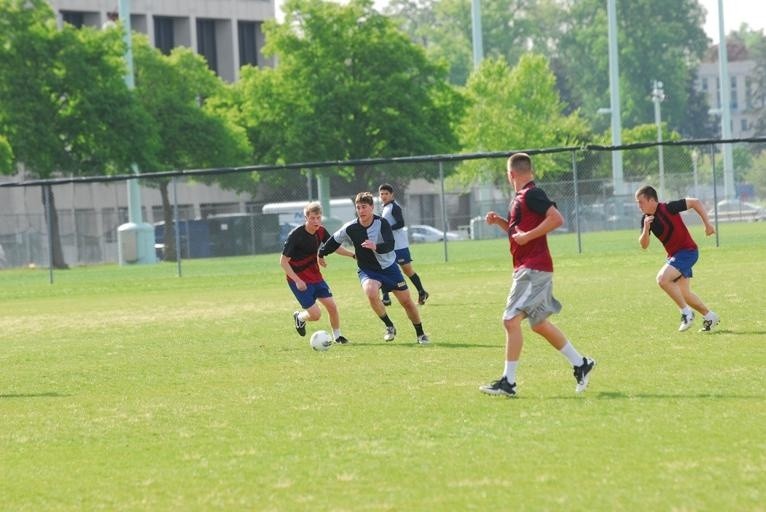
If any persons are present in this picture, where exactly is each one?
[379,183,429,306]
[280,200,357,345]
[635,185,721,332]
[478,153,597,399]
[317,192,431,345]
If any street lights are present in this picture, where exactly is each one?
[691,149,700,199]
[649,73,665,197]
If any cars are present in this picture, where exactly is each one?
[467,197,643,241]
[707,199,764,225]
[409,224,460,243]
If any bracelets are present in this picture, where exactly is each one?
[353,254,355,259]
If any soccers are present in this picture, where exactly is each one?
[311,330,333,351]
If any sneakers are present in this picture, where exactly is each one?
[573,356,596,393]
[479,376,517,397]
[292,310,306,337]
[416,334,432,344]
[333,336,351,344]
[678,310,695,332]
[384,324,397,342]
[698,313,721,332]
[414,289,430,306]
[381,297,392,306]
